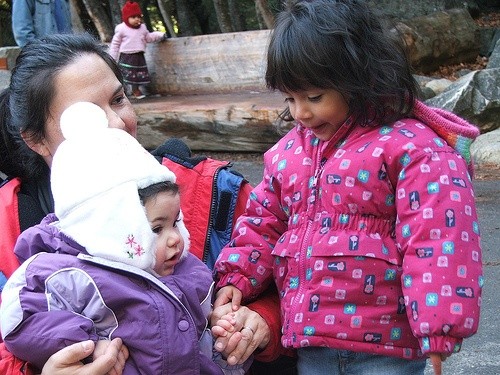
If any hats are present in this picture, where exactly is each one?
[50,102,191,271]
[121,0,142,28]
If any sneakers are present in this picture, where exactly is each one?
[130,88,146,100]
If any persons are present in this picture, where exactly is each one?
[0,31,295,375]
[107,1,168,99]
[212,0,484,375]
[0,102,255,375]
[12,0,72,50]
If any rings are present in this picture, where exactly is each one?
[242,326,255,334]
